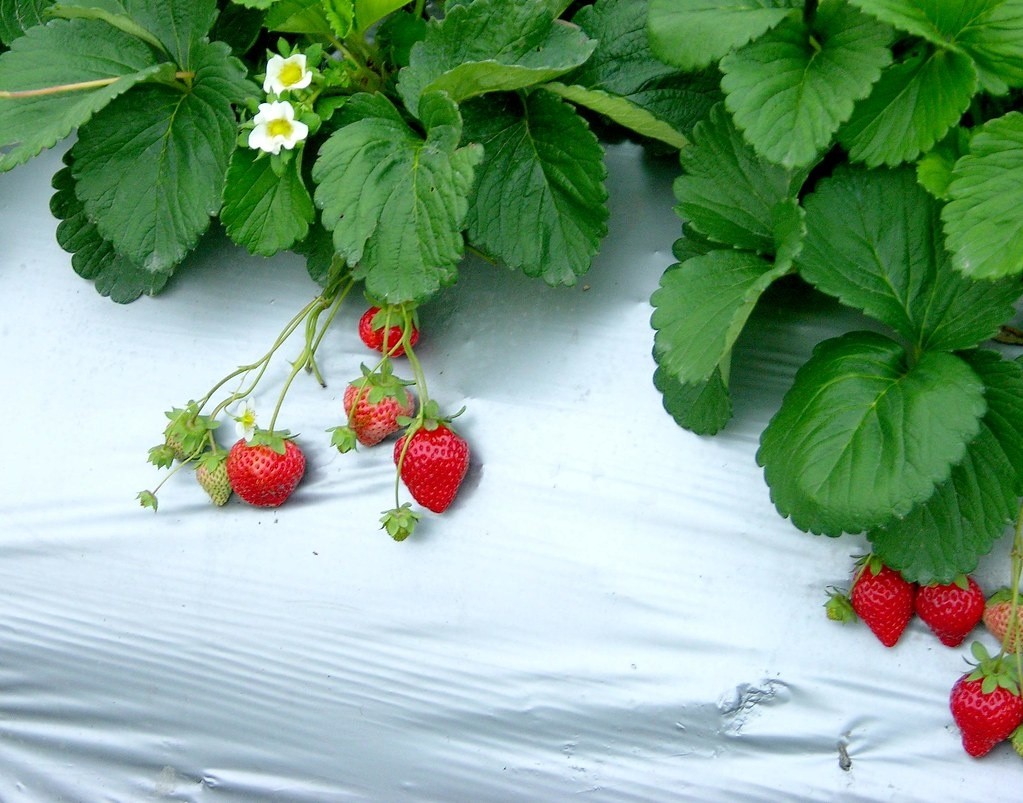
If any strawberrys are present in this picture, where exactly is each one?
[825,554,1023,759]
[164,302,469,542]
